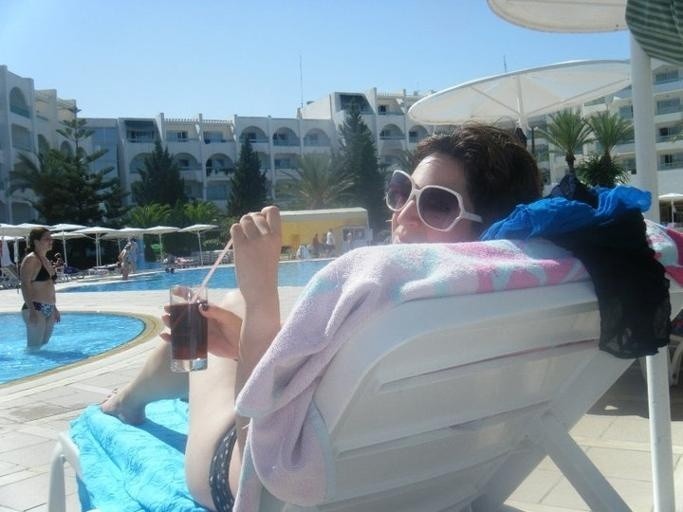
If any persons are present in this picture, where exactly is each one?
[98,121,541,510]
[295,228,353,259]
[18,228,60,350]
[118,243,131,280]
[127,233,139,273]
[51,252,63,270]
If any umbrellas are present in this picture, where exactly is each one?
[487,0,629,34]
[405,57,633,135]
[143,224,179,261]
[657,191,682,227]
[103,226,147,244]
[0,218,120,268]
[177,222,221,266]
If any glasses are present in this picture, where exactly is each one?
[382,167,480,233]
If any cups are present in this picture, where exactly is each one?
[169,286,209,374]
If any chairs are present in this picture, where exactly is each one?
[0,231,304,297]
[47,221,683,512]
